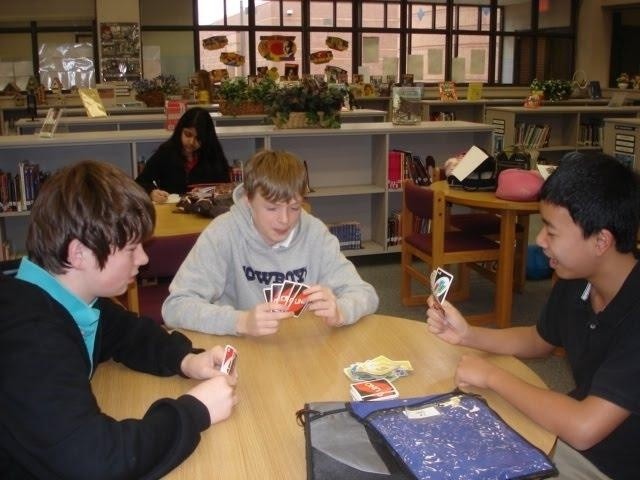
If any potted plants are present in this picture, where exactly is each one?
[617,73,630,89]
[215,75,280,119]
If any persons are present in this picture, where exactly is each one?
[282,40,295,60]
[159,145,382,338]
[0,158,242,480]
[131,106,234,289]
[424,151,640,480]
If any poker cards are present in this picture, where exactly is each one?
[430,267,455,312]
[222,344,239,376]
[350,378,399,401]
[263,280,311,319]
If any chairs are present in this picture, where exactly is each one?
[434,168,529,293]
[112,232,203,323]
[401,179,499,327]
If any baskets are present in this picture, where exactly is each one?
[218,97,269,116]
[274,108,341,131]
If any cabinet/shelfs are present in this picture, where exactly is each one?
[1,85,640,261]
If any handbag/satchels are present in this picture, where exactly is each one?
[298,387,561,480]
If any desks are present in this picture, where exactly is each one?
[432,182,539,327]
[127,197,310,317]
[91,313,558,480]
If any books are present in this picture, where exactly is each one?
[327,207,404,253]
[466,81,484,101]
[277,73,416,112]
[388,111,606,189]
[608,90,629,108]
[0,82,130,113]
[438,80,458,102]
[523,88,545,111]
[1,160,52,267]
[76,86,107,118]
[391,86,422,126]
[589,79,602,100]
[164,99,187,131]
[40,107,61,137]
